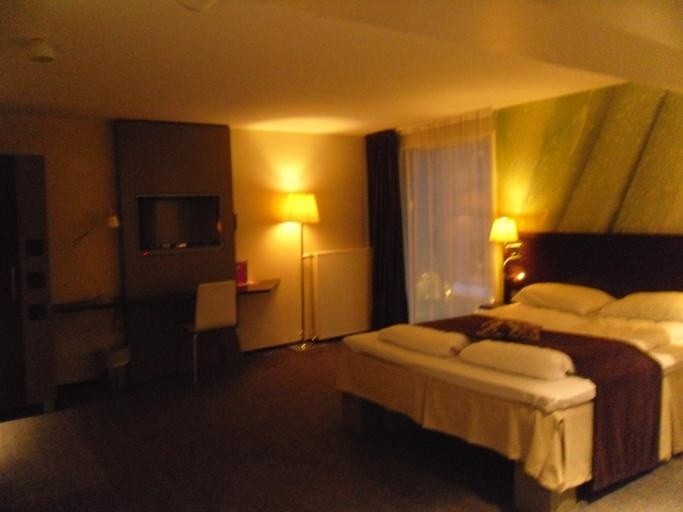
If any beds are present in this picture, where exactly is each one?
[340,230,682,512]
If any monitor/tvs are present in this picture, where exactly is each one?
[135,193,224,257]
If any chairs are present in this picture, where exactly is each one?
[181,278,239,385]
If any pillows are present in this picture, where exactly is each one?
[510,281,682,322]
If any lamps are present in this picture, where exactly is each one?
[281,193,321,352]
[72,205,120,246]
